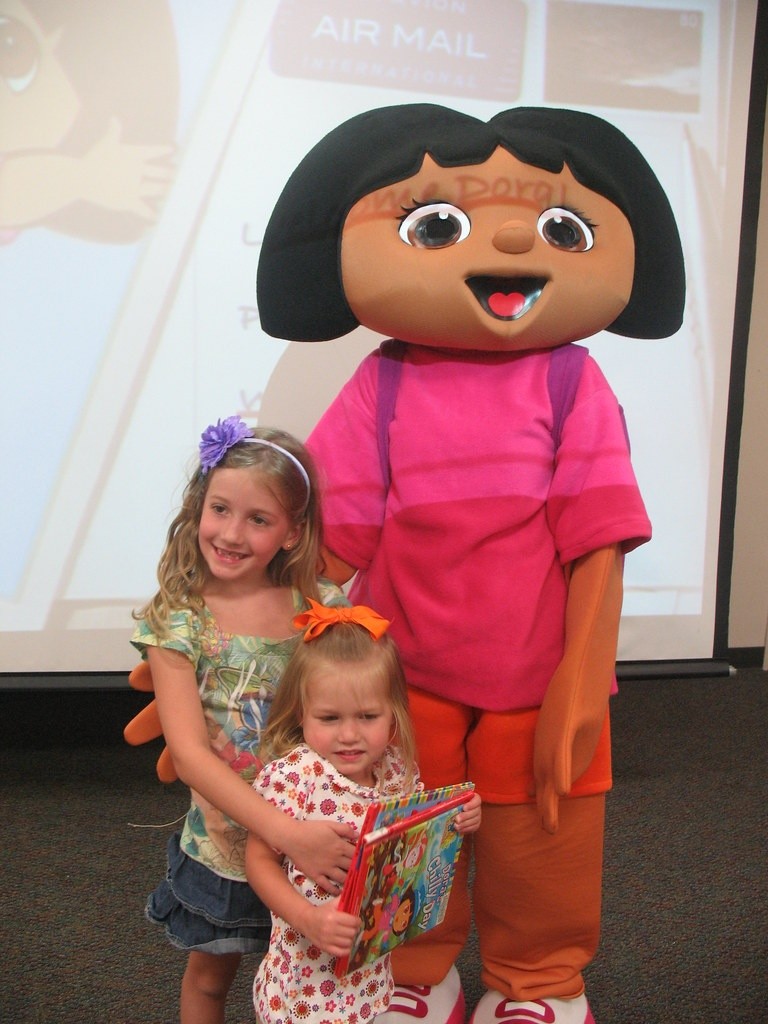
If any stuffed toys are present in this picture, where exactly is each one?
[120,102,686,1024]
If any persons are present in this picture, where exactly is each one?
[130,415,354,1024]
[245,597,483,1024]
